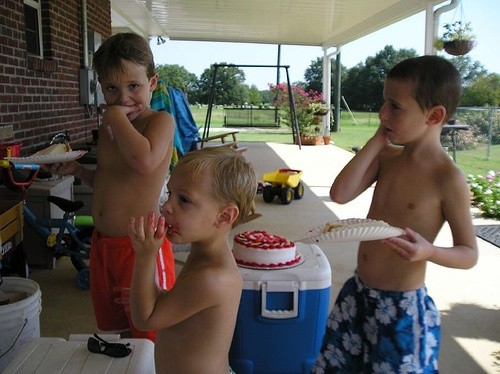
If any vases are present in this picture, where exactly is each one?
[323,134,332,145]
[294,133,321,145]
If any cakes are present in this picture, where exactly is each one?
[324,221,390,232]
[30,143,73,155]
[232,230,300,267]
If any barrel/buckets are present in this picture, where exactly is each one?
[1,276,42,373]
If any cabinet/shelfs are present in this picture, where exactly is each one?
[21,174,76,268]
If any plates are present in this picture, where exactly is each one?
[4,148,87,165]
[293,218,406,244]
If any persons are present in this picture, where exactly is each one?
[90,33,177,332]
[309,54,478,374]
[127,147,258,374]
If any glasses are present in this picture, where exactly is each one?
[87,333,132,359]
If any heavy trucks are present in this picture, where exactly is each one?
[261,167,305,203]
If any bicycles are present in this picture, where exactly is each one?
[0,159,95,275]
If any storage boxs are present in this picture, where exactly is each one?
[228,240,335,374]
[1,329,159,374]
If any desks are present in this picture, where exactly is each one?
[74,161,98,217]
[441,124,469,163]
[0,198,27,279]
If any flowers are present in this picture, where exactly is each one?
[267,80,330,135]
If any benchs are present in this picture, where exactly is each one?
[196,131,239,149]
